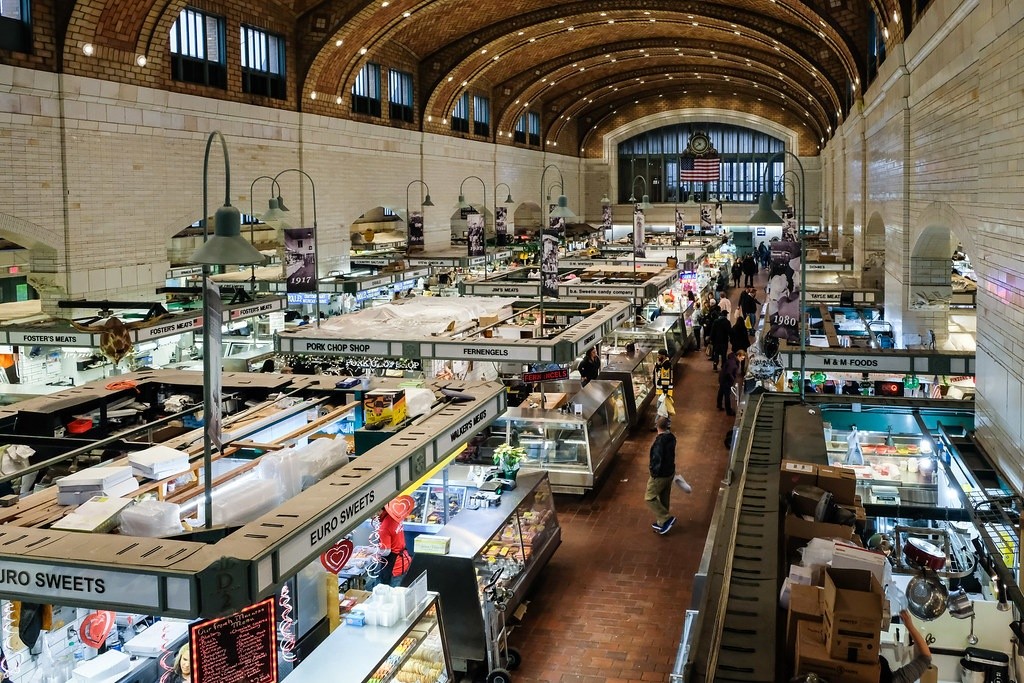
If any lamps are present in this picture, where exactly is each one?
[972,538,1011,611]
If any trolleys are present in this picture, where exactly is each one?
[481,567,522,683]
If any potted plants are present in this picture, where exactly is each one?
[523,243,539,263]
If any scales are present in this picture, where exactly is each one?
[479,482,503,495]
[869,485,901,506]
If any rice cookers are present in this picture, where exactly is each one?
[959,647,1009,683]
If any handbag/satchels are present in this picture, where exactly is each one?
[734,308,740,318]
[673,473,692,494]
[744,315,752,329]
[657,393,674,418]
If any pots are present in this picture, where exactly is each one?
[902,537,947,573]
[944,585,976,620]
[906,572,948,622]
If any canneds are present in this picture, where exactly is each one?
[470,492,489,507]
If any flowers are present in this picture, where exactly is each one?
[492,444,526,471]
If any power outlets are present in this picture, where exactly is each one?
[68,626,75,639]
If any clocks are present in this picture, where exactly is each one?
[690,134,709,154]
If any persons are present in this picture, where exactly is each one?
[165,642,191,683]
[716,349,747,416]
[404,288,415,297]
[362,507,412,588]
[320,311,325,321]
[578,347,601,388]
[690,285,764,371]
[879,609,932,683]
[0,345,19,384]
[650,306,664,321]
[391,291,401,302]
[652,349,674,432]
[645,416,677,535]
[299,315,309,326]
[731,241,770,288]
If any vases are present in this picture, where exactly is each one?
[502,465,520,490]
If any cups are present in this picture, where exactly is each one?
[361,379,370,390]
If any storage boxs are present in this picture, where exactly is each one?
[779,459,891,683]
[384,262,405,271]
[345,610,365,627]
[49,445,190,533]
[339,599,355,614]
[345,589,371,602]
[221,399,234,412]
[365,388,405,427]
[518,392,566,437]
[479,314,498,327]
[68,419,92,433]
[414,534,451,555]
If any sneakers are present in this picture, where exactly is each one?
[651,522,662,530]
[659,516,676,534]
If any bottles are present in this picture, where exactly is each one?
[68,642,77,669]
[469,495,486,508]
[792,372,800,392]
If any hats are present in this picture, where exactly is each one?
[722,310,729,314]
[658,349,670,357]
[749,289,757,292]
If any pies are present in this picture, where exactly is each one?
[398,649,446,683]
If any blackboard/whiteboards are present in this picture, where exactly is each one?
[188,594,279,683]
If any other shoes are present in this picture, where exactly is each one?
[708,358,714,361]
[728,410,735,416]
[695,349,701,352]
[712,364,718,370]
[717,405,724,411]
[750,330,756,336]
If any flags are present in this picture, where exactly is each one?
[681,157,720,181]
